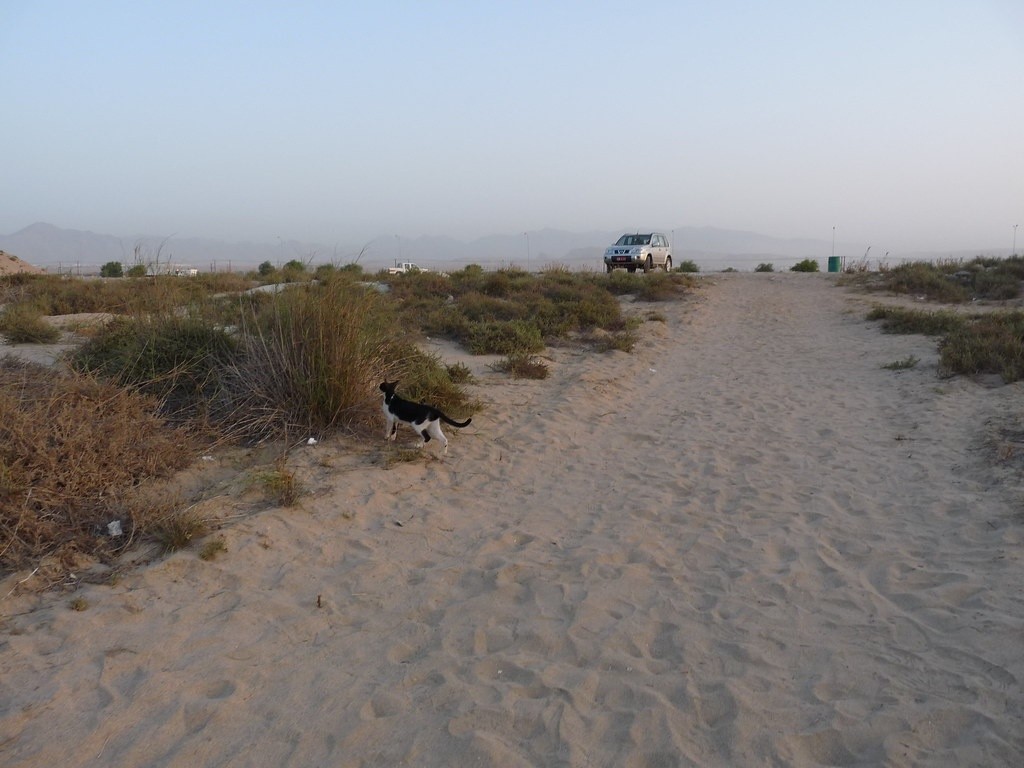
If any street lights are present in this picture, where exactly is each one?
[524,232,531,272]
[277,235,287,271]
[1014,223,1020,256]
[832,226,837,259]
[395,234,402,263]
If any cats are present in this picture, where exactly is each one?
[379,378,473,456]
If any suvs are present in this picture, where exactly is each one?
[604,233,673,272]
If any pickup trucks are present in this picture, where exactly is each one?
[387,262,429,276]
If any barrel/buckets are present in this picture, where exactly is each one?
[828,256,840,272]
